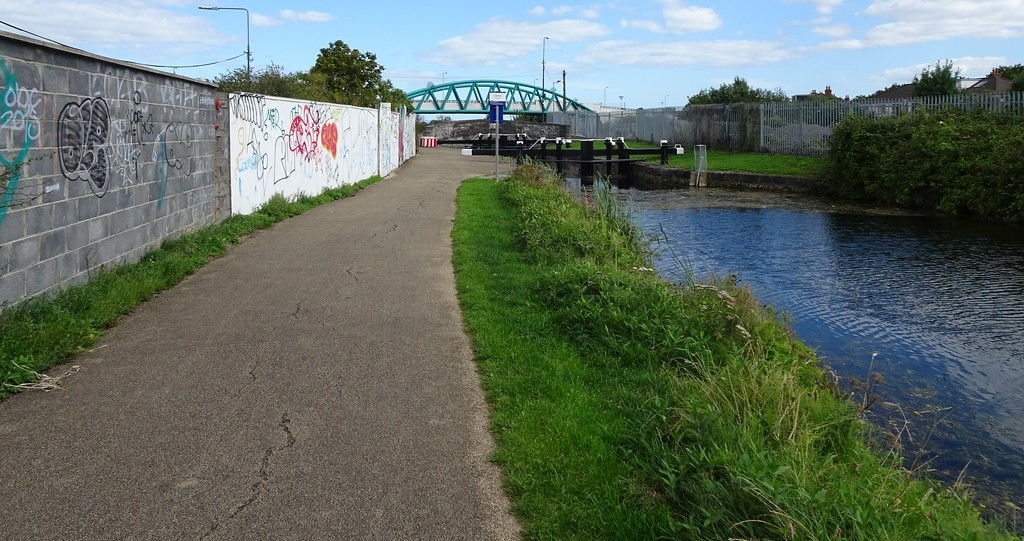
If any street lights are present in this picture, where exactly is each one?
[198,5,250,80]
[604,86,608,107]
[665,95,669,107]
[543,36,549,94]
